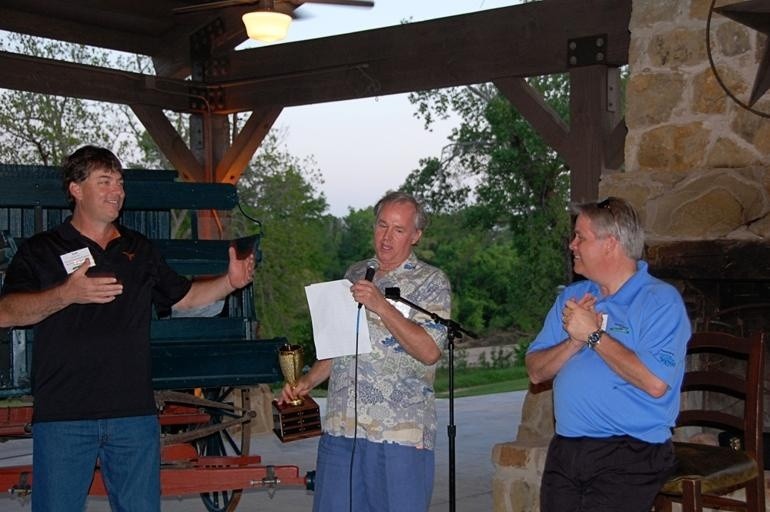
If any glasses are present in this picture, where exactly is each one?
[596,197,618,221]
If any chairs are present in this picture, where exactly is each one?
[653,324,769,511]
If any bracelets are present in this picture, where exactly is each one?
[588,329,606,349]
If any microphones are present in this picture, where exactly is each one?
[357,259,380,309]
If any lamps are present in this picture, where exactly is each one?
[242,1,293,42]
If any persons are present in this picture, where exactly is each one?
[0,144,256,512]
[278,192,453,512]
[525,196,692,511]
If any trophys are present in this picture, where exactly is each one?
[272,344,322,443]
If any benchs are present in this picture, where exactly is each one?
[0,175,291,397]
